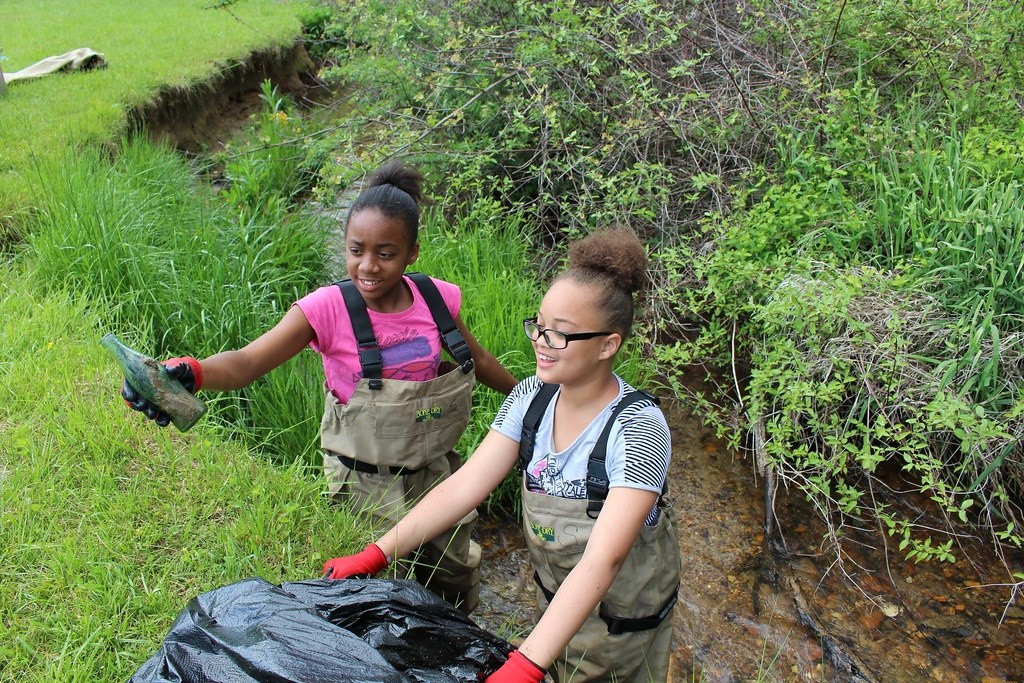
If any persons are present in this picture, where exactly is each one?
[121,162,523,619]
[318,226,681,683]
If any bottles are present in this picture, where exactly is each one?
[99,332,208,433]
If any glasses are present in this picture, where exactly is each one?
[523,316,612,349]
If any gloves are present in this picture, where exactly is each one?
[485,650,548,683]
[120,356,204,427]
[322,542,388,579]
